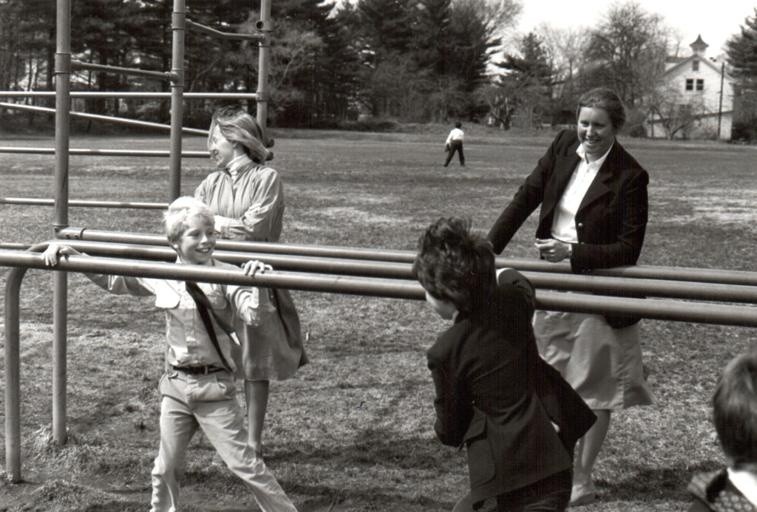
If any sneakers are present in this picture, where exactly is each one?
[568,479,597,507]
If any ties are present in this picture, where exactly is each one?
[184,280,243,375]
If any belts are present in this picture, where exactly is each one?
[165,360,224,376]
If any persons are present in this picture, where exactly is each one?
[40,205,300,512]
[412,217,598,511]
[484,90,651,502]
[443,121,465,168]
[171,112,309,457]
[682,352,757,512]
